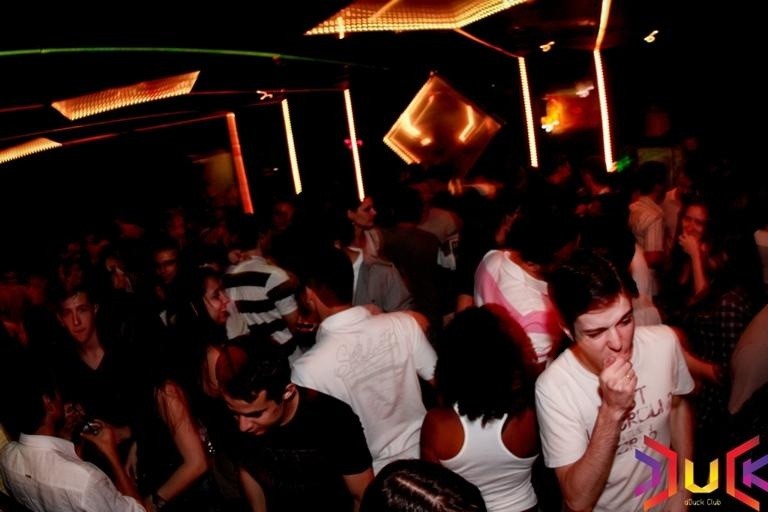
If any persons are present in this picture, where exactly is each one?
[0,108,768,511]
